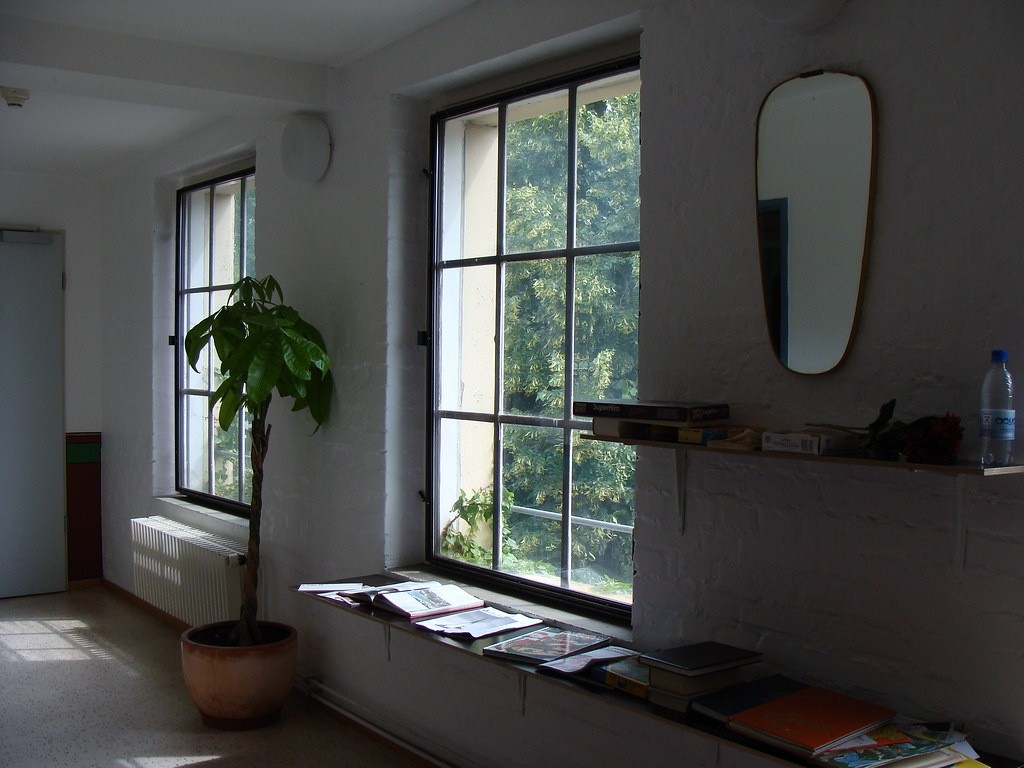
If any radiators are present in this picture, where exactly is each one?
[129,516,249,635]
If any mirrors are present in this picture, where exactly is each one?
[754,69,876,374]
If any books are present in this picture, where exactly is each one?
[297,582,992,768]
[572,401,769,451]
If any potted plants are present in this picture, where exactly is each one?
[180,274,333,737]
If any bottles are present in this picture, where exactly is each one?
[977,347,1016,468]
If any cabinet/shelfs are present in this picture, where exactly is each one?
[289,434,1024,768]
